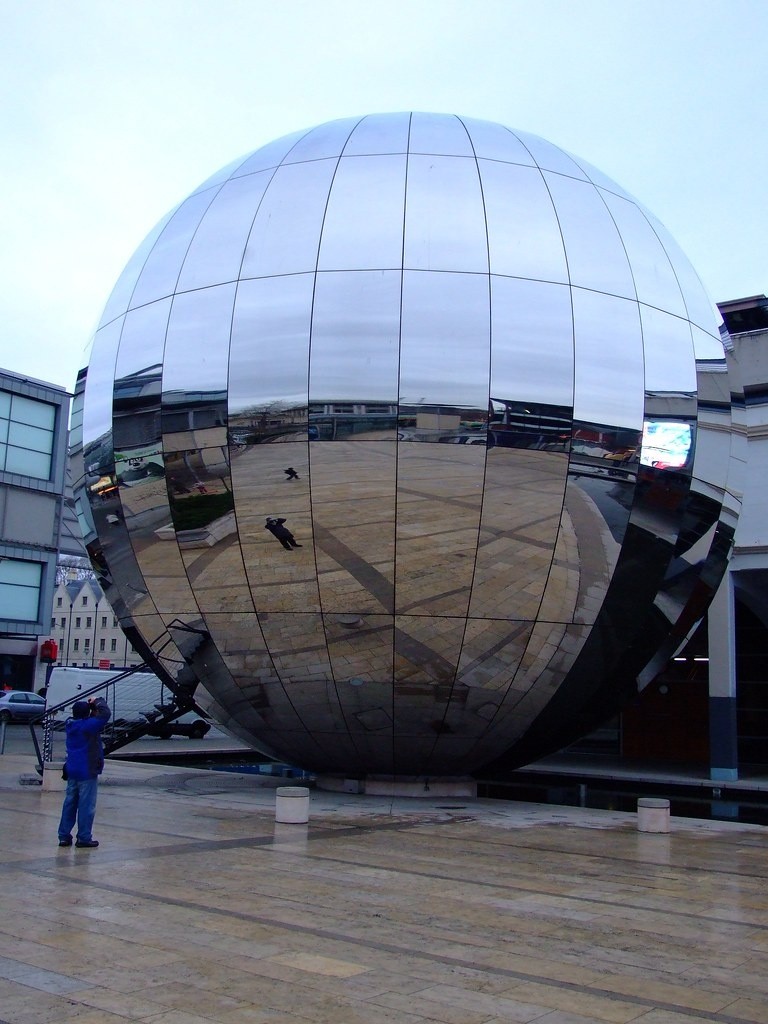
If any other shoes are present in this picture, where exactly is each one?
[76,840,99,847]
[59,839,73,846]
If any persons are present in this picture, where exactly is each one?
[196,479,208,494]
[285,467,301,480]
[105,513,120,526]
[58,697,111,848]
[265,517,303,550]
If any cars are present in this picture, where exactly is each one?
[0,691,48,723]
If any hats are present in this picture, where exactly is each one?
[73,701,90,715]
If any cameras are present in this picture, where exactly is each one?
[270,521,274,524]
[88,700,98,711]
